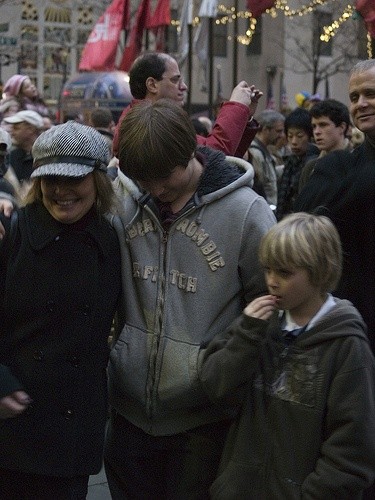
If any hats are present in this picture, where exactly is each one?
[29,119,111,180]
[2,109,45,130]
[3,74,31,98]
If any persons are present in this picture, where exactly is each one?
[0,103,278,500]
[295,59,375,358]
[0,121,122,500]
[0,53,356,240]
[201,213,375,500]
